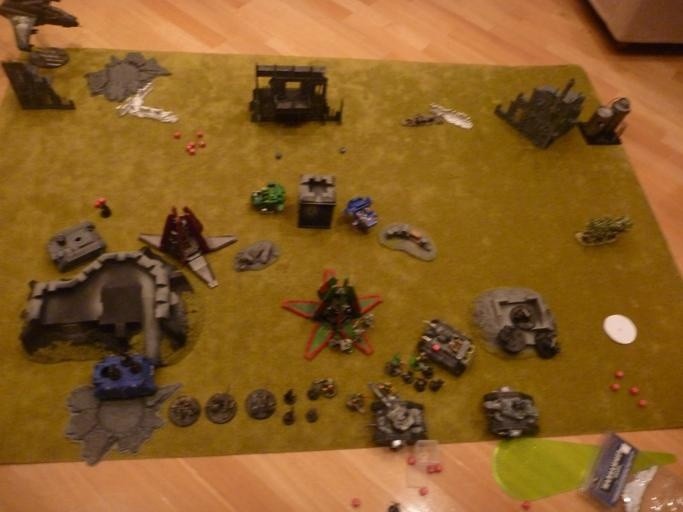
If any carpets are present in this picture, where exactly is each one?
[0,48,683,464]
